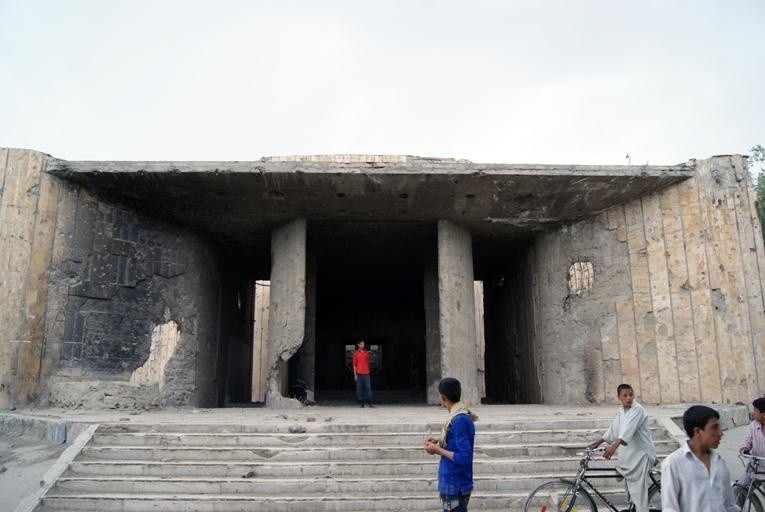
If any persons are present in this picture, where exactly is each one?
[732,398,765,509]
[352,341,377,408]
[660,406,741,512]
[424,378,475,512]
[586,384,656,512]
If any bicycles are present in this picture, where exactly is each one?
[730,450,764,511]
[524,447,661,511]
